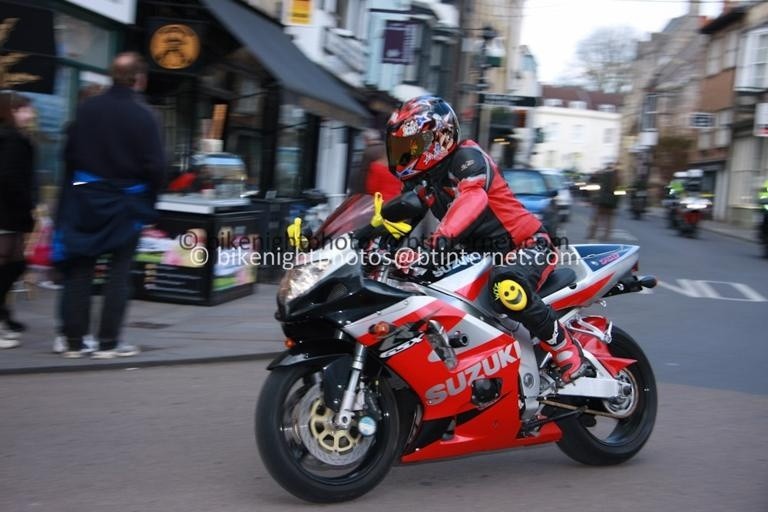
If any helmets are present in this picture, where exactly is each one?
[386,96,462,180]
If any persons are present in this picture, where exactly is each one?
[759,180,767,256]
[59,49,165,358]
[1,92,45,348]
[349,92,586,384]
[663,167,712,237]
[51,86,98,352]
[584,157,620,242]
[627,173,648,216]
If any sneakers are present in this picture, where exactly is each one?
[53,334,139,359]
[0,320,25,348]
[540,324,587,383]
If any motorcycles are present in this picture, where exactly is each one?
[663,193,711,236]
[253,186,658,500]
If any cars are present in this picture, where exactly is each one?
[539,172,572,221]
[501,169,558,239]
